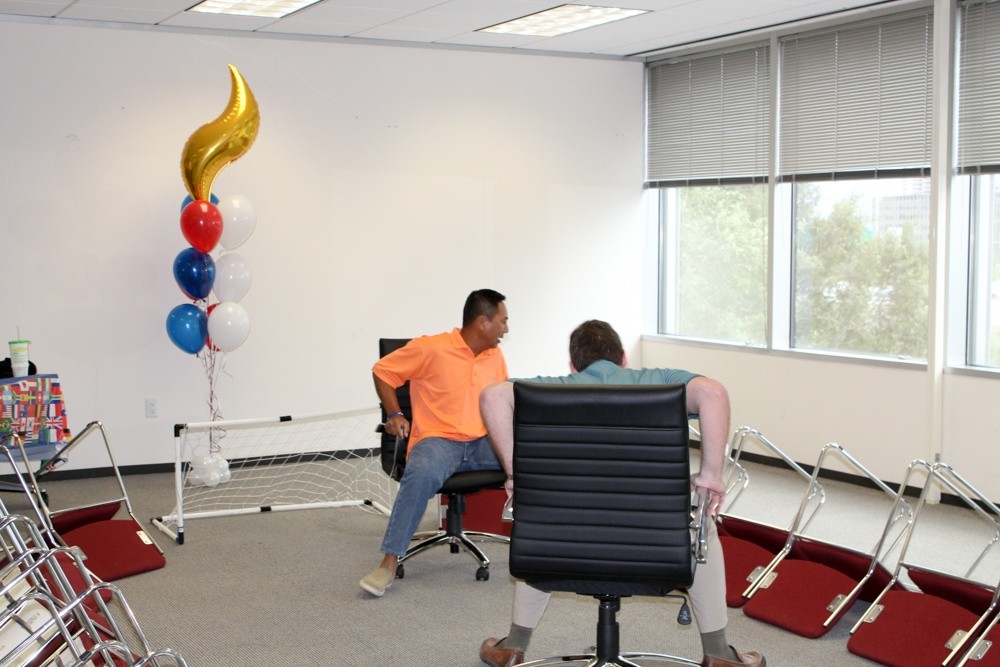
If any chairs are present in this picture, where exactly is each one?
[1,421,194,667]
[377,340,999,667]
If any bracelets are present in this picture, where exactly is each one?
[505,473,513,480]
[387,412,404,420]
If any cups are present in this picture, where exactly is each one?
[9,340,29,378]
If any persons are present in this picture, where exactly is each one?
[478,319,767,667]
[357,289,514,598]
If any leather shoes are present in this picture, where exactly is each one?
[701,644,766,667]
[479,637,524,667]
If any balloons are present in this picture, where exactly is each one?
[160,61,261,357]
[187,453,231,486]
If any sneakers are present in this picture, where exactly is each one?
[359,566,396,597]
